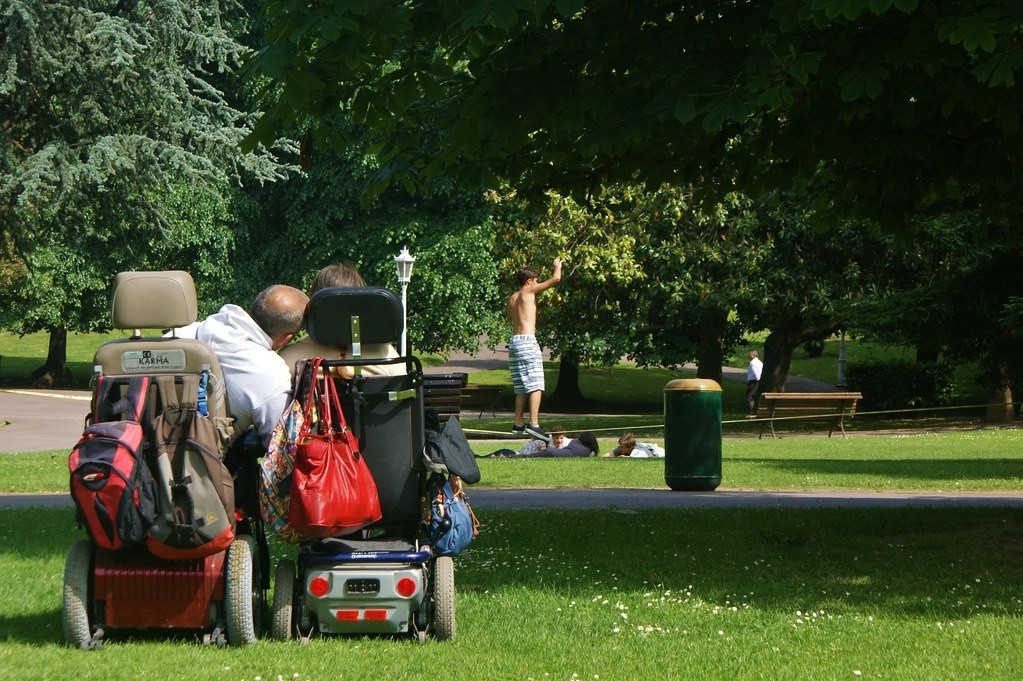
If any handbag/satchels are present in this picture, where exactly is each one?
[427,473,479,556]
[288,356,383,538]
[425,415,481,485]
[256,359,322,542]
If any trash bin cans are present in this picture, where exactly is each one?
[662,376,725,493]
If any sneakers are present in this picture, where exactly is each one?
[525,423,549,441]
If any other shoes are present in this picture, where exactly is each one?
[512,423,526,432]
[745,412,758,419]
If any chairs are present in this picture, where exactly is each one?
[94,270,229,428]
[294,287,425,530]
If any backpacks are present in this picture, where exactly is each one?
[68,376,161,552]
[145,376,236,560]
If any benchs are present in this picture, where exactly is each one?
[461,385,508,419]
[427,375,465,423]
[746,392,863,439]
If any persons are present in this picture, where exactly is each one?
[746,351,763,418]
[278,265,408,383]
[508,258,563,442]
[162,285,310,629]
[474,426,600,458]
[603,433,665,458]
